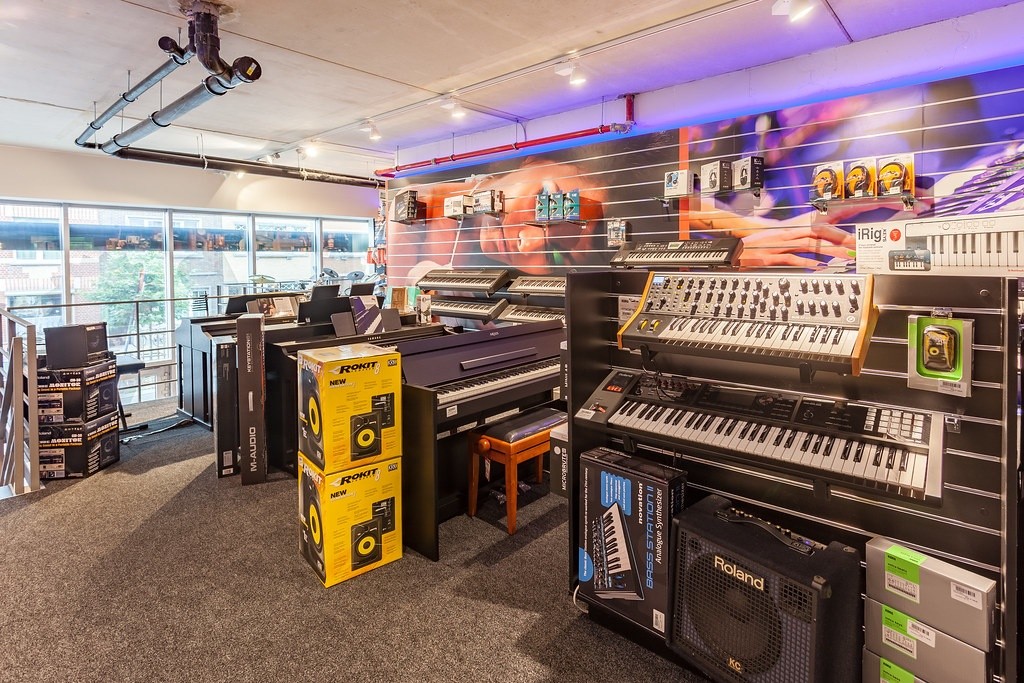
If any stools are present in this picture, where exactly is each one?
[470,407,568,534]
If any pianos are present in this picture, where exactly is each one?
[170,313,242,432]
[415,268,511,294]
[609,236,746,267]
[506,275,567,294]
[396,318,570,563]
[497,303,567,326]
[571,365,950,506]
[265,321,460,478]
[413,295,509,325]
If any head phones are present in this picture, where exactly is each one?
[709,172,717,189]
[666,172,678,184]
[740,169,747,186]
[813,162,910,195]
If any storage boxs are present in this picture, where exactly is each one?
[298,453,404,588]
[535,193,549,221]
[296,346,403,475]
[565,189,581,221]
[663,170,693,197]
[700,162,733,193]
[39,313,269,485]
[548,191,565,220]
[444,195,476,216]
[855,212,1024,278]
[474,190,504,212]
[860,534,998,682]
[393,190,418,220]
[733,156,765,190]
[573,451,687,639]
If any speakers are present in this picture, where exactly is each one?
[671,495,858,683]
[98,380,119,468]
[44,322,109,370]
[298,369,396,583]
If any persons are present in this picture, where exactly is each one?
[387,151,623,285]
[688,184,934,272]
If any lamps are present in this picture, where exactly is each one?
[554,59,587,86]
[770,0,816,23]
[439,98,465,118]
[360,123,381,140]
[266,154,281,163]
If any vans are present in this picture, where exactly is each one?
[6,293,75,355]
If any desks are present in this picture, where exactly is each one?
[116,356,149,434]
